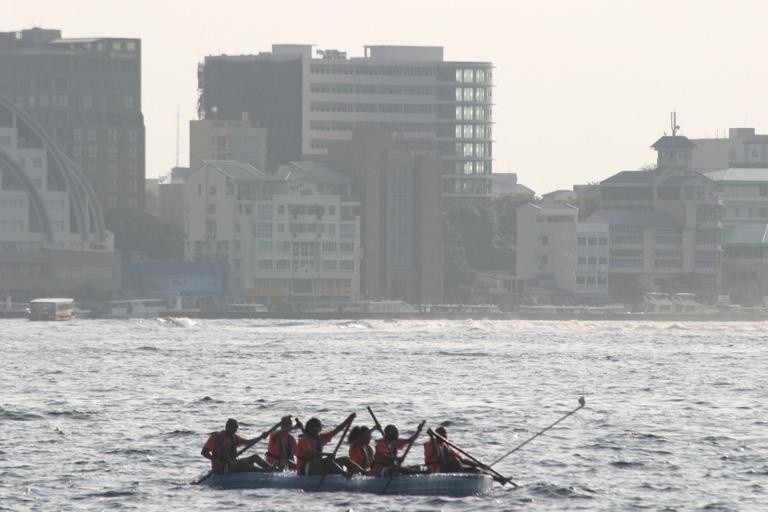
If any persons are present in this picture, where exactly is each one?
[346,423,381,475]
[200,417,281,475]
[370,423,427,477]
[294,412,374,479]
[424,425,484,475]
[265,414,304,469]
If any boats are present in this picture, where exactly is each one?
[22,297,77,320]
[208,468,495,498]
